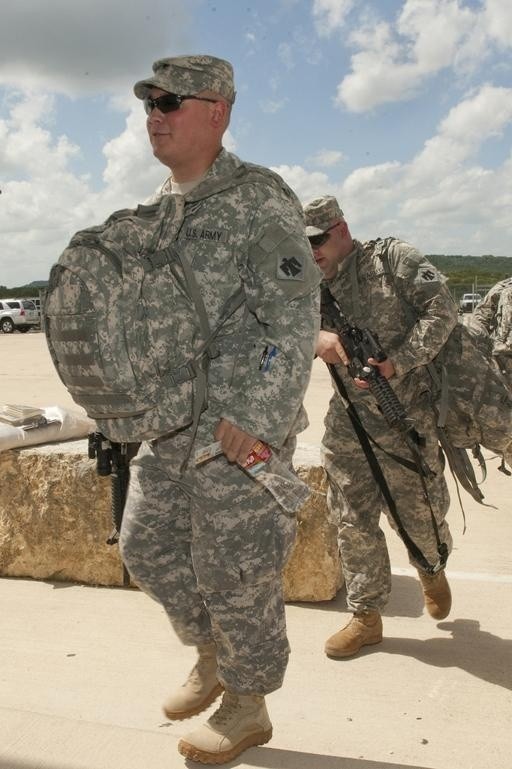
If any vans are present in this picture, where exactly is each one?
[28,299,40,329]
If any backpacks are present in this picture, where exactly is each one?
[412,308,512,504]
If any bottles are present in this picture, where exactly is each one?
[238,436,315,514]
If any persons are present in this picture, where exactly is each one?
[298,192,463,659]
[120,53,325,767]
[469,272,512,355]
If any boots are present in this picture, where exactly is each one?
[164,642,224,720]
[418,568,451,620]
[178,690,273,765]
[325,605,382,657]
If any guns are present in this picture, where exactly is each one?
[89,431,142,585]
[321,281,436,481]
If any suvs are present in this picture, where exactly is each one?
[0,298,38,334]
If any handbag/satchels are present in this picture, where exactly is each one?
[43,195,215,444]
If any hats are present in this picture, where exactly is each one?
[303,196,343,237]
[133,55,236,102]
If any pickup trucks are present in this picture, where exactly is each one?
[460,293,483,314]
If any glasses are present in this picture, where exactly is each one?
[145,94,215,114]
[308,223,341,248]
